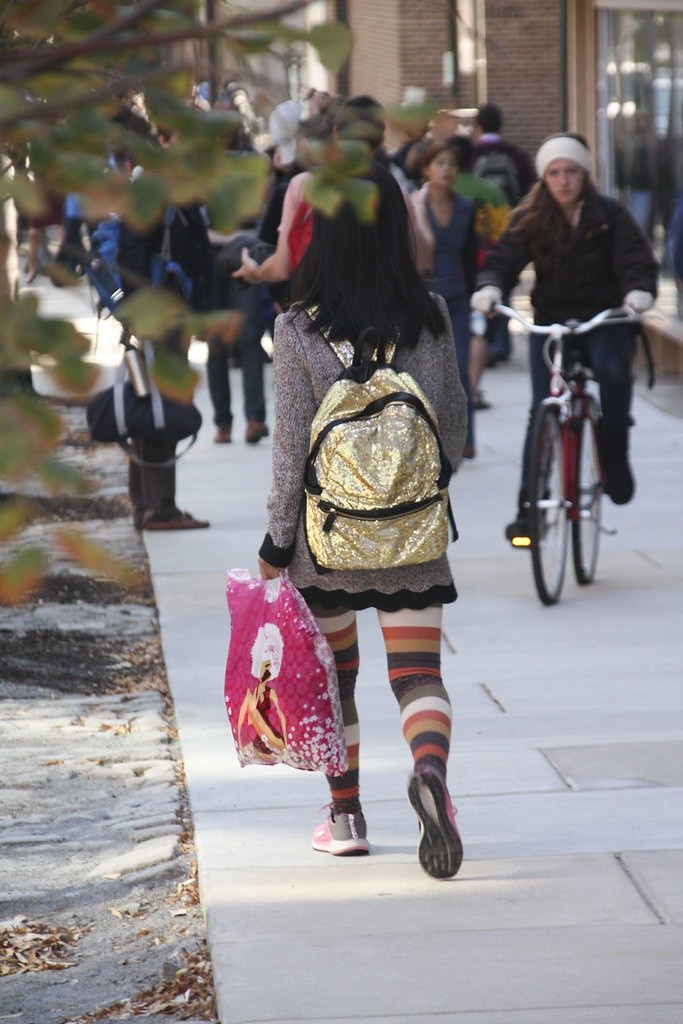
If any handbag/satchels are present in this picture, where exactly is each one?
[87,343,203,469]
[225,571,350,778]
[148,253,192,351]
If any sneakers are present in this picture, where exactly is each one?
[408,764,463,879]
[311,802,370,855]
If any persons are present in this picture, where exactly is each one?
[474,130,660,545]
[251,166,469,880]
[39,66,537,531]
[609,111,683,319]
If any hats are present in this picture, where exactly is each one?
[534,132,592,178]
[269,100,303,164]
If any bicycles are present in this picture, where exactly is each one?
[472,299,653,608]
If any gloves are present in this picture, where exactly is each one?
[624,290,654,311]
[470,286,503,318]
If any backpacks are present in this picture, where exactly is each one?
[302,306,458,575]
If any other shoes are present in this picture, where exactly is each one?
[245,420,269,442]
[214,427,231,443]
[506,523,529,540]
[604,472,633,505]
[144,511,210,530]
